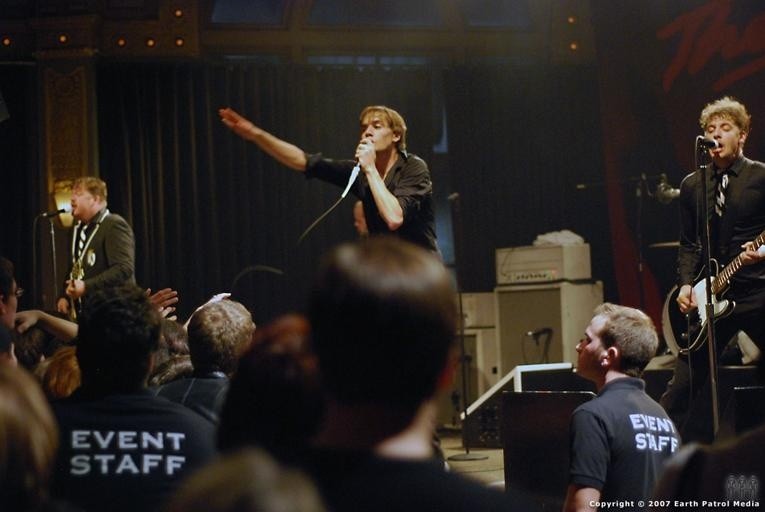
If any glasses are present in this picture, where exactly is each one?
[2,285,23,298]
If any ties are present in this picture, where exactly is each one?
[77,224,88,260]
[713,173,729,220]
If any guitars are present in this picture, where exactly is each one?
[67,260,79,321]
[667,235,765,353]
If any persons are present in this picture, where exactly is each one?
[54,176,139,324]
[218,104,444,264]
[560,301,684,512]
[657,94,763,416]
[1,236,538,512]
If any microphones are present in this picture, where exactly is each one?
[654,182,680,206]
[698,135,718,150]
[43,206,70,216]
[354,158,362,175]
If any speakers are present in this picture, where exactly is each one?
[712,386,764,445]
[438,327,496,430]
[697,363,764,426]
[493,280,605,380]
[499,390,595,492]
